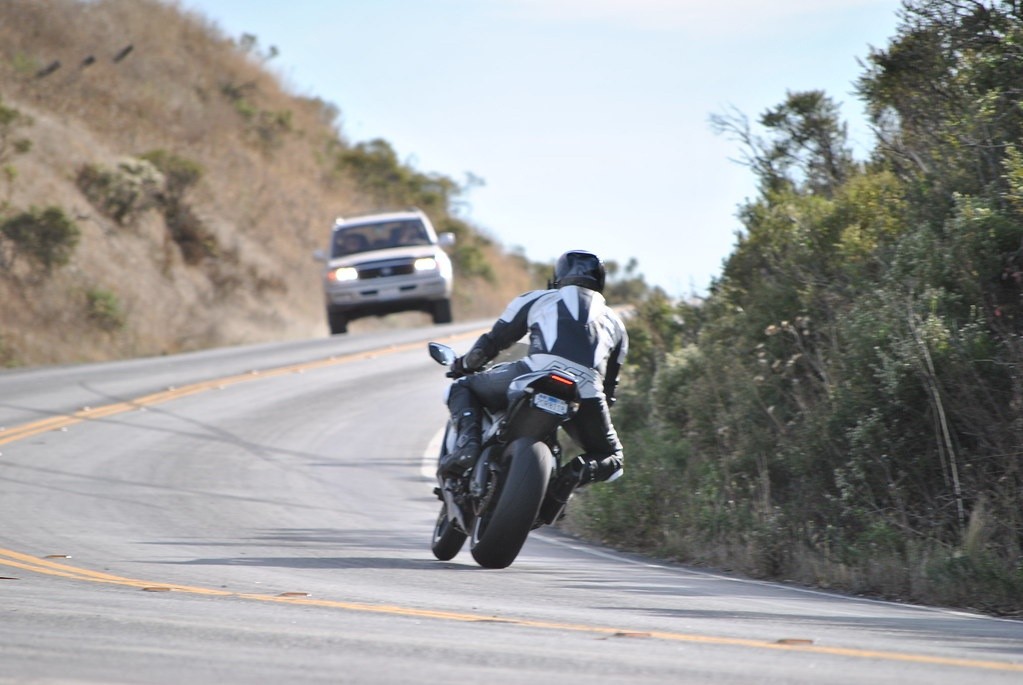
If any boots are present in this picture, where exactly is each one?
[577,452,599,488]
[434,405,482,473]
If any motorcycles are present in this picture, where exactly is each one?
[425,338,592,568]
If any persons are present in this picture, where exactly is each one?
[438,247,628,521]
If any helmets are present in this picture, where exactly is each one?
[547,248,607,293]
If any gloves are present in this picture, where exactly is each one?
[447,333,497,379]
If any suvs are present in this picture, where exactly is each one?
[310,204,457,334]
